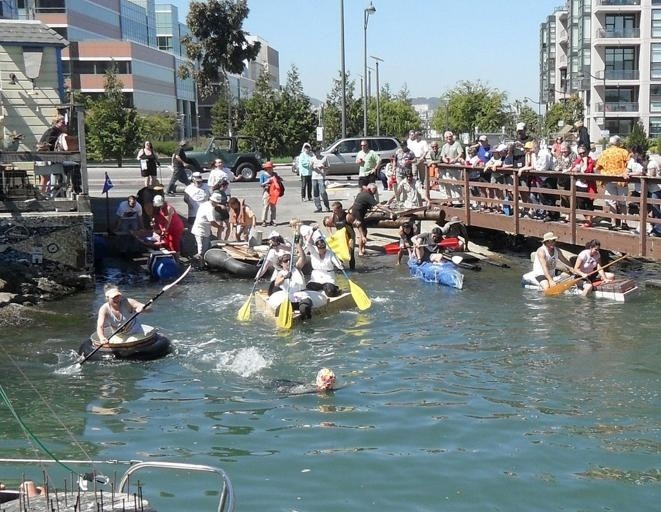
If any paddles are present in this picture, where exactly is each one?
[237,242,274,321]
[58,264,191,374]
[385,238,461,255]
[217,235,284,246]
[546,254,629,298]
[278,221,297,330]
[316,223,373,311]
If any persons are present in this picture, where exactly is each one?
[260,161,278,227]
[165,140,192,195]
[97,281,153,348]
[113,158,256,257]
[299,143,330,212]
[315,367,336,390]
[35,115,66,191]
[356,127,661,237]
[346,183,469,264]
[137,141,160,186]
[533,232,611,298]
[253,202,355,317]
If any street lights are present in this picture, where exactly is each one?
[521,96,542,139]
[358,55,383,102]
[549,88,566,143]
[576,70,606,149]
[362,5,377,136]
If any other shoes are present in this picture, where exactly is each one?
[440,201,560,222]
[561,217,659,236]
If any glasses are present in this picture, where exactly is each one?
[283,259,290,263]
[193,176,201,178]
[361,143,367,146]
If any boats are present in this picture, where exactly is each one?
[0,343,236,512]
[255,281,359,328]
[77,323,170,360]
[96,230,182,284]
[408,253,466,293]
[322,205,447,229]
[520,266,639,303]
[201,237,292,277]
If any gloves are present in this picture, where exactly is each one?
[294,235,300,243]
[284,272,292,279]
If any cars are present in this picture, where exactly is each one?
[461,131,526,169]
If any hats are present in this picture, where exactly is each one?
[540,232,557,243]
[609,135,620,144]
[523,142,534,148]
[264,162,272,167]
[210,193,222,203]
[449,216,463,225]
[312,229,325,244]
[193,172,202,182]
[303,144,310,147]
[479,136,487,141]
[517,122,525,131]
[104,289,122,299]
[268,231,279,238]
[400,140,407,146]
[153,195,163,206]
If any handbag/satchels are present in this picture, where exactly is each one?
[268,182,285,197]
[268,177,280,205]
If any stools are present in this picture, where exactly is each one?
[5,169,27,185]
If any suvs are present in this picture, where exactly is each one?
[291,137,403,185]
[176,133,261,182]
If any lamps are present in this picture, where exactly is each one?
[22,52,42,82]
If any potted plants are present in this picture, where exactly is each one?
[2,130,25,151]
